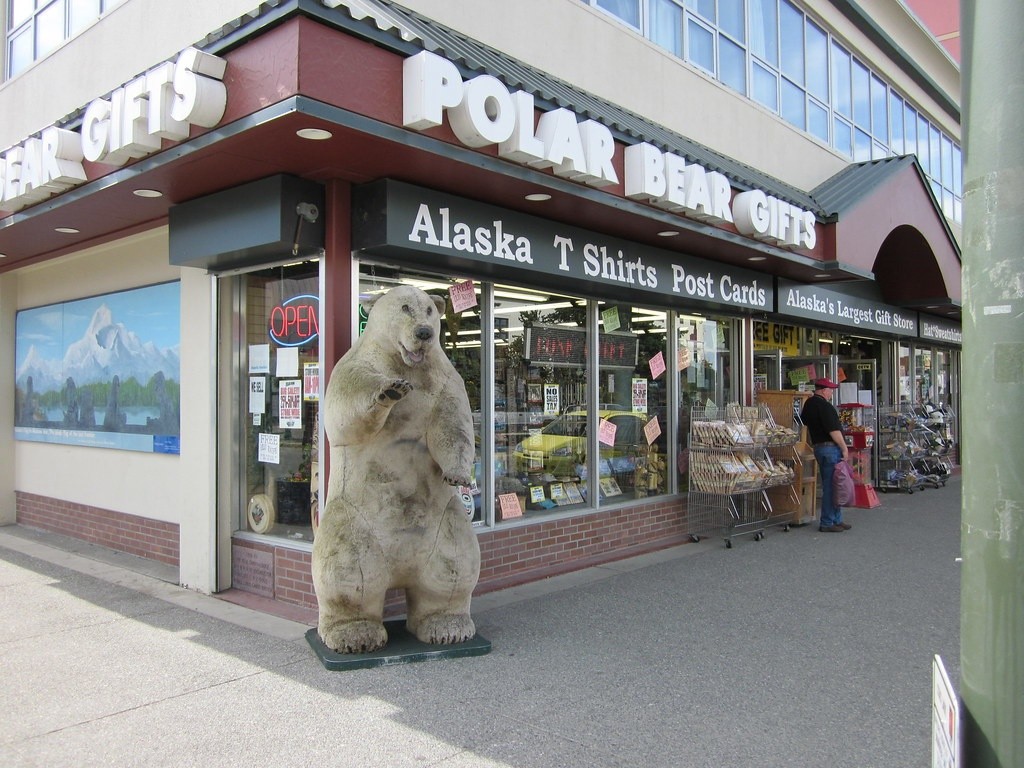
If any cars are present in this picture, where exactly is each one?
[512,413,650,475]
[528,415,547,427]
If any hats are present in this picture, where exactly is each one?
[815,378,838,388]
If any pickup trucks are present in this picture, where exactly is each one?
[471,400,539,430]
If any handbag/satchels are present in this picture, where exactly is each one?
[832,460,856,506]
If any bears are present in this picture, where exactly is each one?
[308,283,482,657]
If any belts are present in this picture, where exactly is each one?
[814,442,835,447]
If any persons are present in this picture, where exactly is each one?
[800,377,851,533]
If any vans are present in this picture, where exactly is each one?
[564,403,624,414]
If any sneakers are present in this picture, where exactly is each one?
[820,524,843,532]
[833,522,851,529]
